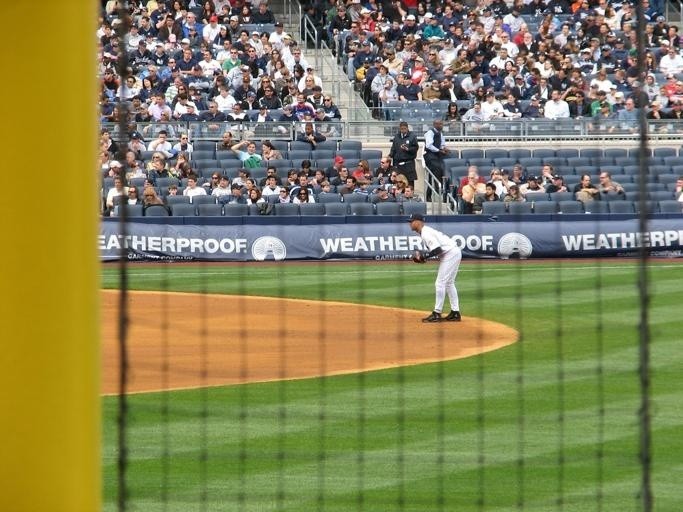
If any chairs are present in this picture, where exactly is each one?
[93,0,682,234]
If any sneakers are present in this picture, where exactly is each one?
[439,310,462,321]
[422,311,441,322]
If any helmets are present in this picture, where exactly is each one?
[247,90,256,97]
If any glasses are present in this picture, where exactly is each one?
[231,52,236,54]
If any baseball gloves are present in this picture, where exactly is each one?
[413,250,427,264]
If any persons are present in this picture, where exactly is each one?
[406,214,462,322]
[95,1,682,218]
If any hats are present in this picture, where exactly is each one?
[130,132,139,140]
[529,175,539,181]
[484,91,495,98]
[448,22,456,27]
[656,16,665,23]
[429,15,439,19]
[182,38,191,44]
[335,156,344,163]
[230,16,239,22]
[412,56,423,63]
[168,34,177,43]
[315,108,325,113]
[274,21,283,27]
[607,31,616,37]
[405,213,424,221]
[498,169,508,174]
[580,48,592,53]
[156,42,165,49]
[194,64,202,71]
[231,183,243,190]
[475,49,485,57]
[552,175,563,179]
[460,33,470,41]
[361,40,371,47]
[210,16,218,22]
[602,45,611,50]
[491,64,498,71]
[406,15,416,21]
[237,166,251,175]
[241,66,252,82]
[139,39,148,46]
